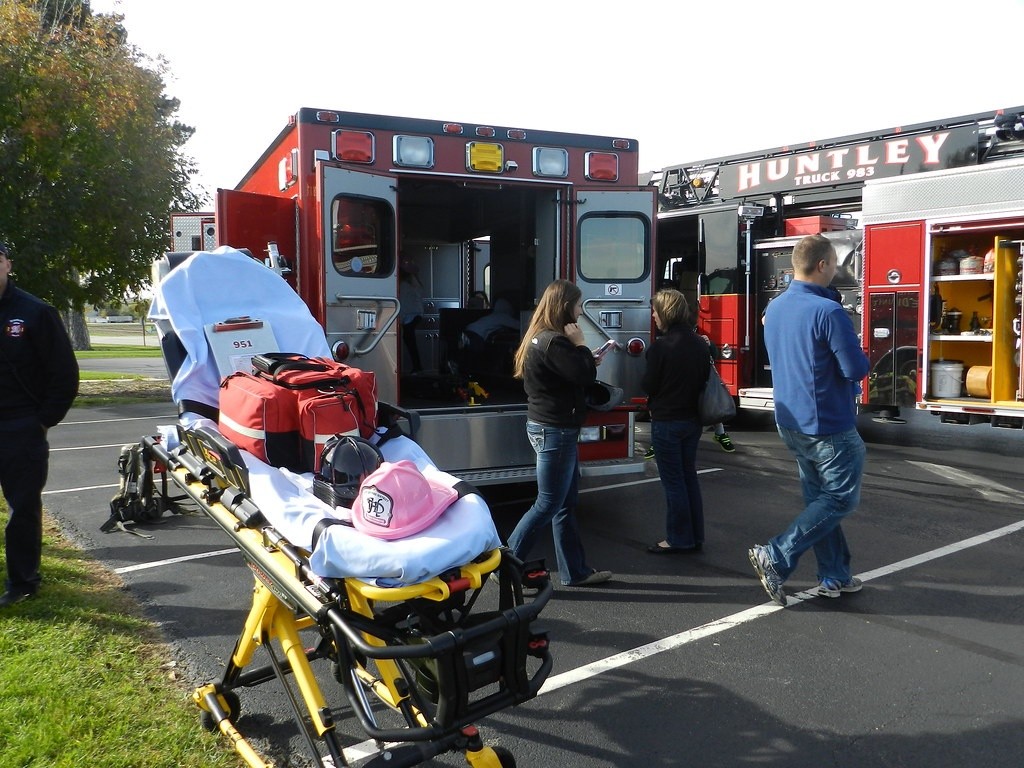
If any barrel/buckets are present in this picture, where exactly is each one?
[930,359,965,397]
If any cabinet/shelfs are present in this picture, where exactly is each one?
[926,224,997,405]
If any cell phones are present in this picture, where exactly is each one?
[593,340,617,359]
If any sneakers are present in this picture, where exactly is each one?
[746,542,793,608]
[712,431,735,452]
[643,444,655,459]
[815,571,866,598]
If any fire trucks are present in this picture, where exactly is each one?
[633,103,1023,431]
[170,106,648,495]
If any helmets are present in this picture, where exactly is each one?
[350,458,458,540]
[313,432,390,508]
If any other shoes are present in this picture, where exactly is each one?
[646,538,706,555]
[0,591,34,608]
[490,569,538,595]
[561,568,612,586]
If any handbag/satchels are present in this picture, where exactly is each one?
[218,353,422,475]
[700,360,737,426]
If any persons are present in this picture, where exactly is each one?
[489,280,613,593]
[646,288,710,556]
[449,298,520,374]
[643,300,735,459]
[0,241,80,610]
[399,268,424,375]
[748,235,871,606]
